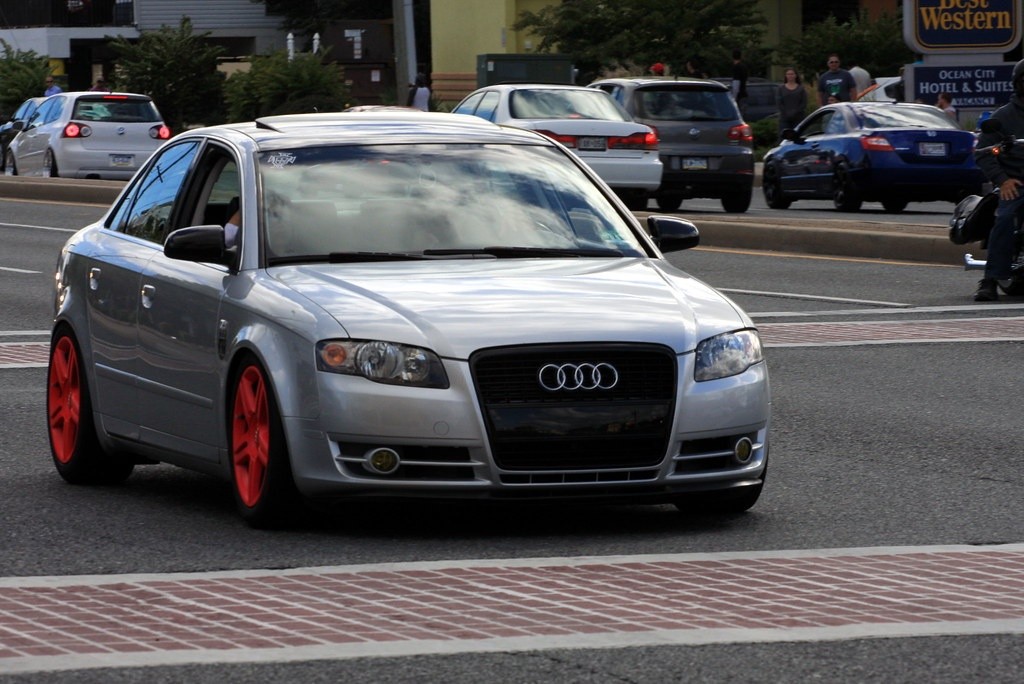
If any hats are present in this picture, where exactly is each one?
[96,75,104,81]
[650,63,664,74]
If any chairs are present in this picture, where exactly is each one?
[204,199,454,262]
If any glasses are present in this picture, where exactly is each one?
[829,61,839,64]
[46,81,52,83]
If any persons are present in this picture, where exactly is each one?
[974,58,1024,301]
[845,58,871,96]
[44,76,62,97]
[407,73,431,112]
[913,94,935,105]
[892,67,905,103]
[775,67,807,147]
[224,190,308,256]
[938,93,958,121]
[817,53,857,105]
[732,47,749,102]
[91,76,111,92]
[686,59,703,79]
[649,63,665,76]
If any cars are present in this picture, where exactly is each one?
[763,100,979,216]
[47,109,772,525]
[1,91,174,179]
[449,83,662,210]
[584,76,757,212]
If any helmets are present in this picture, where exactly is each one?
[1011,59,1024,96]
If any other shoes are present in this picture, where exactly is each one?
[974,279,999,301]
